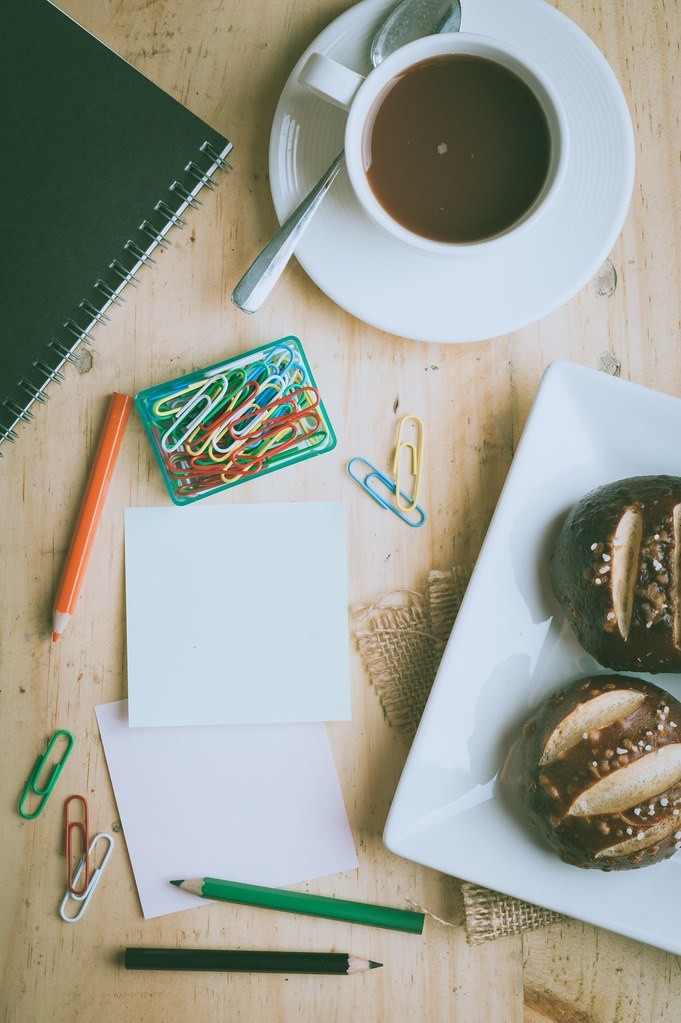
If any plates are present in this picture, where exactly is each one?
[269,0,636,343]
[382,355,681,958]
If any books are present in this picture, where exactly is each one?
[0,0,232,457]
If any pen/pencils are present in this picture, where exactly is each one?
[127,946,384,975]
[53,393,135,643]
[167,878,426,936]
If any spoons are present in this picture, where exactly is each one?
[229,0,462,315]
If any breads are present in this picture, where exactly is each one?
[518,672,681,872]
[548,474,681,675]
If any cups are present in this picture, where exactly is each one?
[297,31,570,256]
